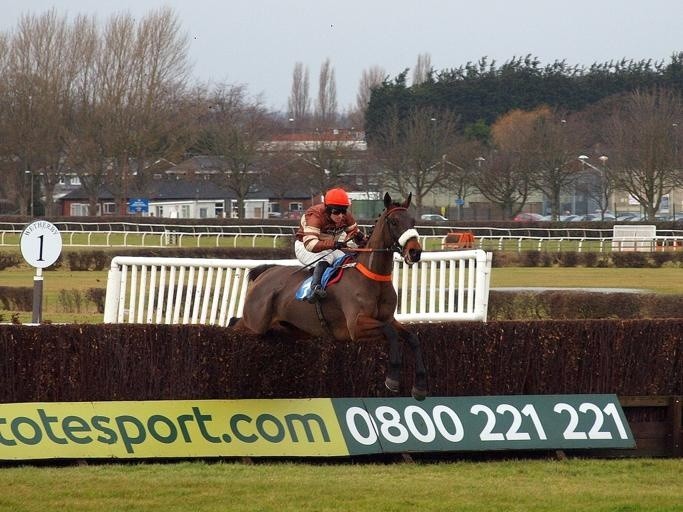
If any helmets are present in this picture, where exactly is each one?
[324,187,350,208]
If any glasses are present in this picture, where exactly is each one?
[326,206,346,216]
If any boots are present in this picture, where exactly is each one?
[311,261,330,299]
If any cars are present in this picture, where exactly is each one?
[419,208,682,227]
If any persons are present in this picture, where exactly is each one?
[294,186,369,297]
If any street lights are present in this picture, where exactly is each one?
[23,170,43,221]
[577,153,609,209]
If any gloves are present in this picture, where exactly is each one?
[336,241,347,250]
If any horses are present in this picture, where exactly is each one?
[226,191,428,402]
